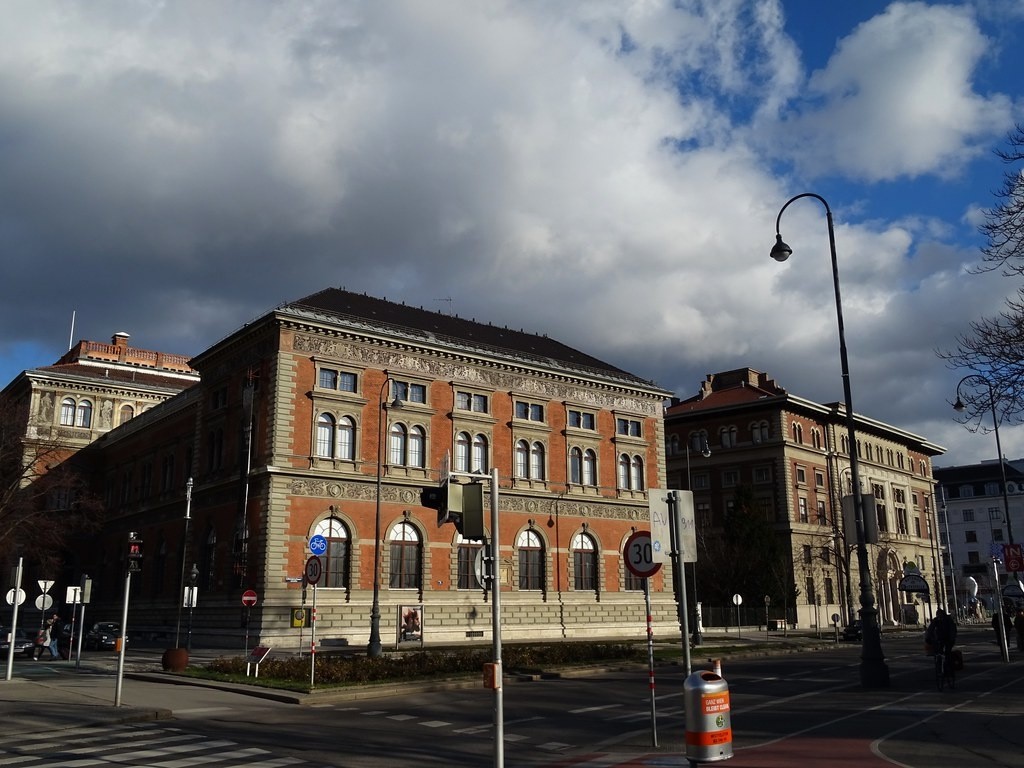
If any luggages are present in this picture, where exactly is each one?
[929,655,948,692]
[57,646,73,660]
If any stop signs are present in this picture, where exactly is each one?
[242,590,258,607]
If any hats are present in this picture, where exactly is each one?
[47,618,53,625]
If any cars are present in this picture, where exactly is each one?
[61,623,87,649]
[841,619,882,642]
[0,628,36,658]
[83,622,129,650]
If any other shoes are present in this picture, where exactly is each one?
[48,656,57,661]
[33,656,40,661]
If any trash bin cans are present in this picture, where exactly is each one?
[35,628,46,646]
[683,670,734,763]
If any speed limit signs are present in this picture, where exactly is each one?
[305,556,322,585]
[623,531,663,577]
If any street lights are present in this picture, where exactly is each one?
[367,377,402,658]
[771,193,890,690]
[161,477,193,673]
[114,531,143,707]
[955,374,1018,580]
[683,430,712,647]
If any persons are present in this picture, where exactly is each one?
[101,400,113,429]
[969,602,1024,656]
[40,392,53,422]
[926,609,958,658]
[33,614,73,661]
